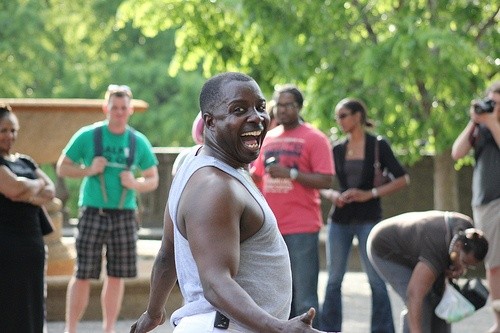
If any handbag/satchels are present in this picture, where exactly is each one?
[449,274,490,310]
[37,206,54,235]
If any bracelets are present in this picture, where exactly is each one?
[290,168,298,181]
[372,188,377,197]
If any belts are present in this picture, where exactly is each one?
[82,207,134,216]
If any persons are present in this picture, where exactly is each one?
[56,85,159,333]
[319,97,410,333]
[451,82,500,333]
[250,87,336,332]
[366,210,489,333]
[130,72,325,333]
[0,104,56,333]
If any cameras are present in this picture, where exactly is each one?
[474,98,496,114]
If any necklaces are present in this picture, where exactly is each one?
[348,150,355,155]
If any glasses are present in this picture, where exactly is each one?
[333,111,356,121]
[460,252,475,270]
[108,84,131,93]
